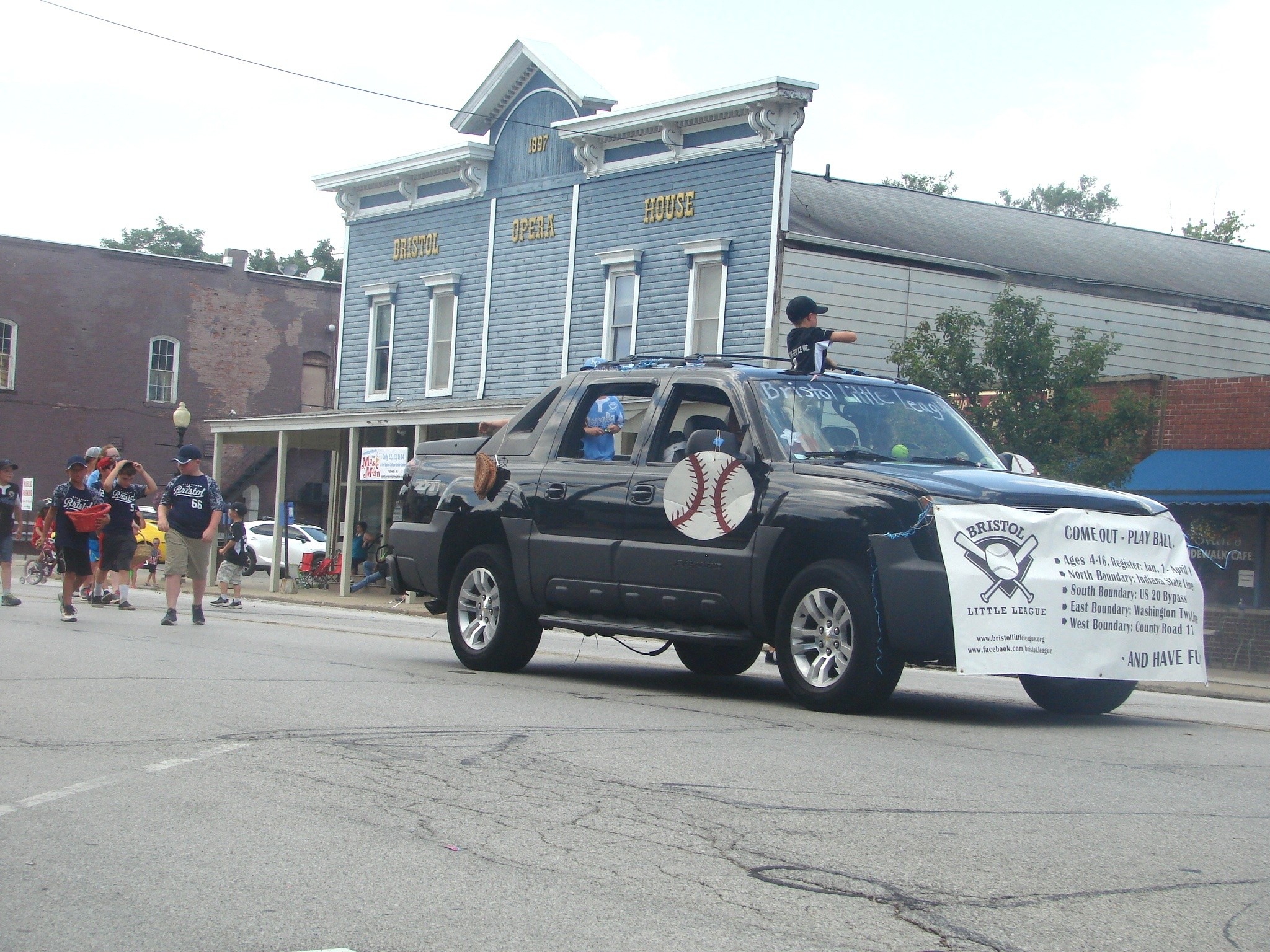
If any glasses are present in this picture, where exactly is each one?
[154,543,160,544]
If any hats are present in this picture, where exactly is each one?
[358,521,367,531]
[580,357,607,370]
[86,446,102,459]
[113,458,134,469]
[225,501,247,516]
[787,296,828,322]
[0,459,18,470]
[96,457,120,469]
[170,444,201,464]
[66,455,88,470]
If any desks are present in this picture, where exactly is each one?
[1225,616,1263,671]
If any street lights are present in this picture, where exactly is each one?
[174,401,191,476]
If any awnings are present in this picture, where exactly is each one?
[1103,449,1270,504]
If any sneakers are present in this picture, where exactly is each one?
[210,597,230,606]
[58,591,65,614]
[1,592,21,606]
[60,605,77,622]
[161,608,178,625]
[192,605,205,624]
[229,599,242,609]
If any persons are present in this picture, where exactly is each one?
[210,502,248,609]
[31,445,158,611]
[0,459,22,606]
[350,561,388,593]
[157,444,224,625]
[580,356,624,461]
[786,296,858,376]
[144,538,163,587]
[478,419,510,434]
[350,521,375,583]
[35,455,112,622]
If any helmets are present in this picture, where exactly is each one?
[39,497,53,510]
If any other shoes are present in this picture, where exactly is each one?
[34,559,43,572]
[146,582,151,586]
[367,582,376,586]
[153,583,159,586]
[72,584,136,611]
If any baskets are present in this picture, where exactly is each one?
[129,532,152,570]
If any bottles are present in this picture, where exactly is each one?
[1238,598,1244,617]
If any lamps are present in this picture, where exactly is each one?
[325,324,336,333]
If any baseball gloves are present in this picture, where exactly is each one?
[474,452,497,500]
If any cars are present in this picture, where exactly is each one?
[241,516,328,579]
[134,519,166,564]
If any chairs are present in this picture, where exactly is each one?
[327,553,342,582]
[819,427,859,449]
[298,553,313,588]
[662,415,730,463]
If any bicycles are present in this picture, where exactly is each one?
[19,545,66,585]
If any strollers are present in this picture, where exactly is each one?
[299,548,341,589]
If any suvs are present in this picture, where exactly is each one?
[378,353,1194,715]
